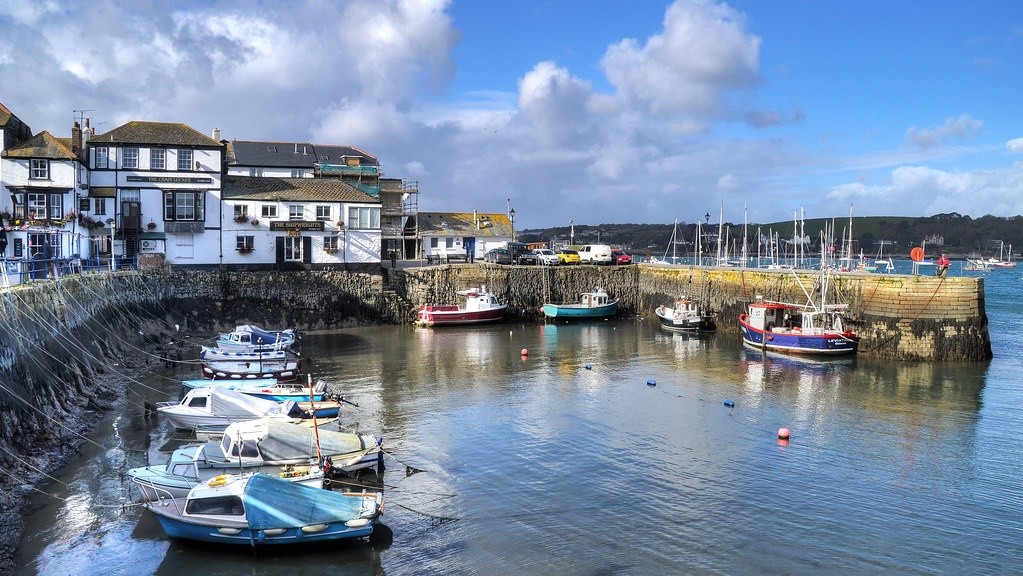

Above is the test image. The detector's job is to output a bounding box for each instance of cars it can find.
[530,248,560,266]
[483,248,512,266]
[554,249,582,265]
[605,249,632,265]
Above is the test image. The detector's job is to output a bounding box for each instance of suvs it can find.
[507,242,537,266]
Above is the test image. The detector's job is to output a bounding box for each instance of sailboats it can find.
[961,239,1018,272]
[648,196,892,272]
[915,239,936,266]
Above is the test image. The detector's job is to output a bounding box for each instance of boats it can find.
[414,286,510,327]
[125,322,387,505]
[542,287,622,319]
[738,230,860,357]
[132,429,385,547]
[654,297,717,333]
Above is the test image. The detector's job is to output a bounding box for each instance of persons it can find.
[390,251,396,267]
[939,253,949,278]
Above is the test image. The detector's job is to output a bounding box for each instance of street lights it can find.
[704,211,710,267]
[509,208,516,267]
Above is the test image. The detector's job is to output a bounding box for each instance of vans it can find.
[576,244,613,265]
